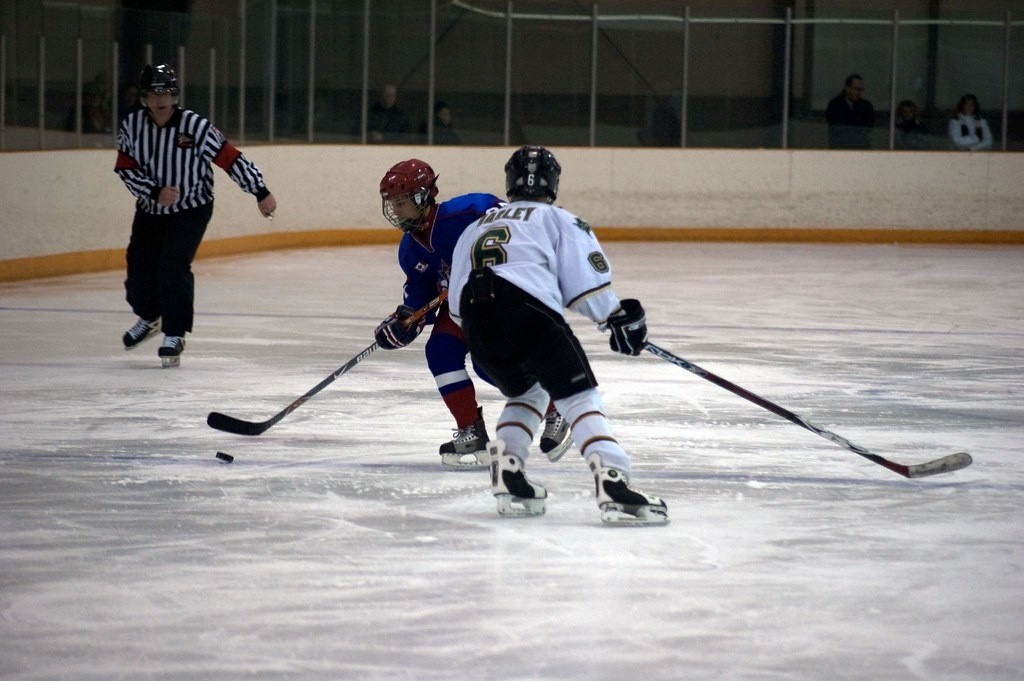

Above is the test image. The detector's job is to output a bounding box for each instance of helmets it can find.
[380,159,439,211]
[505,145,562,203]
[137,64,179,96]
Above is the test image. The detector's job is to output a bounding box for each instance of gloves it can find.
[374,304,426,351]
[607,297,648,357]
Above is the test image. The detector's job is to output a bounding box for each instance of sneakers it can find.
[485,439,548,498]
[122,317,161,347]
[538,410,571,454]
[585,452,668,513]
[158,336,186,356]
[439,406,491,455]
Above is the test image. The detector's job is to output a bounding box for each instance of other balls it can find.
[216,452,234,462]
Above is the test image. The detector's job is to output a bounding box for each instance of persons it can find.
[447,146,669,527]
[355,85,463,144]
[949,93,993,151]
[114,64,277,368]
[372,159,573,467]
[890,101,930,149]
[827,73,874,149]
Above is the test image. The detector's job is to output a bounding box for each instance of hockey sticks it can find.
[644,343,972,478]
[206,288,448,436]
